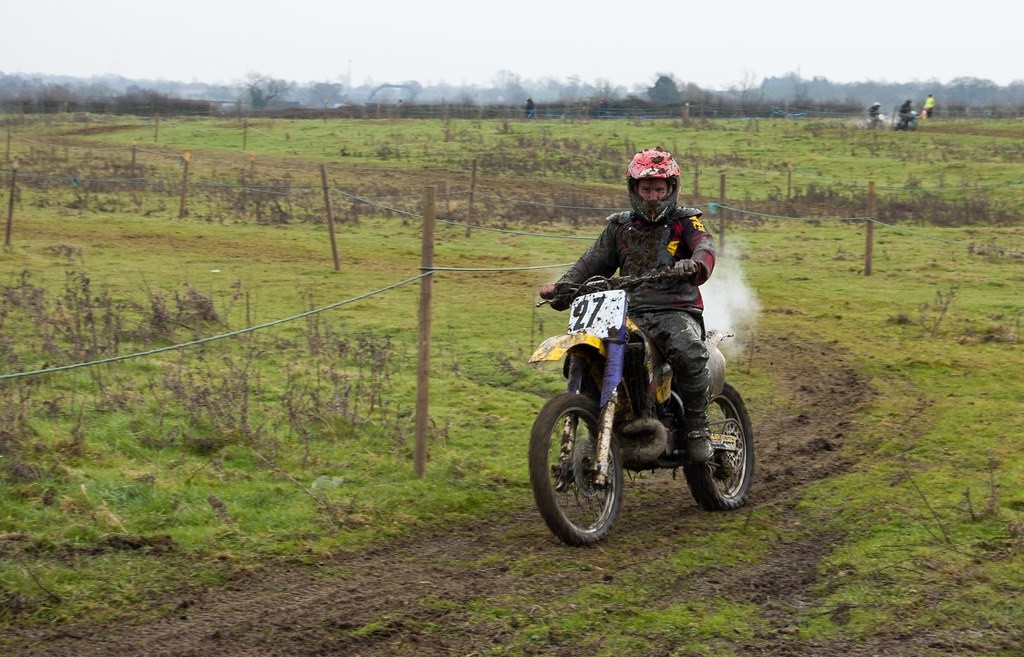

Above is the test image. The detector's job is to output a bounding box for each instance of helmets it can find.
[625,147,680,223]
[907,101,911,103]
[874,102,881,106]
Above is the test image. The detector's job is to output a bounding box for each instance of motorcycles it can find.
[893,108,917,131]
[769,104,806,118]
[866,112,889,130]
[528,265,755,545]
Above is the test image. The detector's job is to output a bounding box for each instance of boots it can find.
[685,399,715,463]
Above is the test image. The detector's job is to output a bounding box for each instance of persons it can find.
[868,102,880,122]
[599,98,609,117]
[898,100,912,126]
[539,145,716,463]
[525,98,535,118]
[923,95,935,118]
